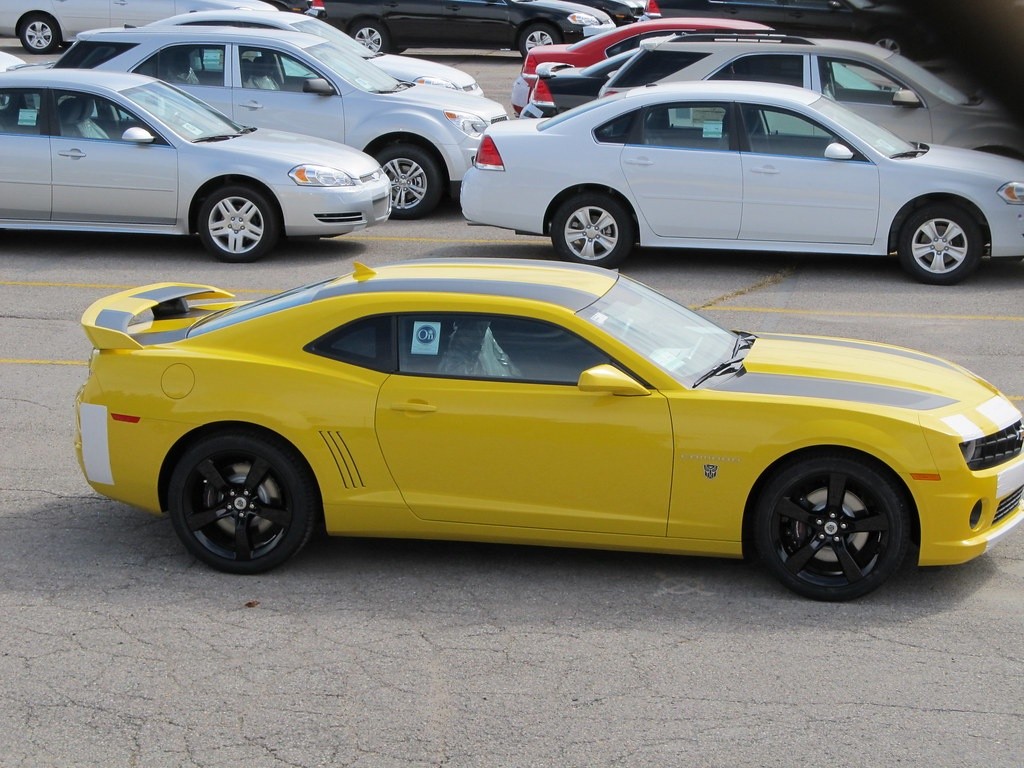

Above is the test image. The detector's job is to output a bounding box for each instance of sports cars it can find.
[74,260,1024,603]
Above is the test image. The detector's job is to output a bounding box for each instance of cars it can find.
[266,0,645,55]
[0,0,278,55]
[512,0,979,124]
[146,10,484,155]
[460,81,1024,285]
[0,69,392,263]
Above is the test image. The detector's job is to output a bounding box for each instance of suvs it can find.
[50,24,507,219]
[596,32,1024,161]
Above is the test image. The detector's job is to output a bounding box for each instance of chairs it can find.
[646,110,672,131]
[241,57,260,89]
[58,96,111,140]
